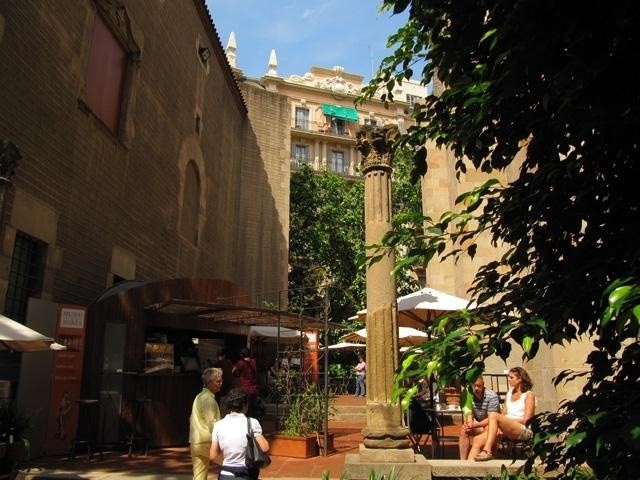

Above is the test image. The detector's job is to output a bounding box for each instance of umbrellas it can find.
[319,327,439,352]
[0,315,68,355]
[348,286,512,459]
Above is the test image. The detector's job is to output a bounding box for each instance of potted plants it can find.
[273,365,337,454]
[263,382,321,457]
[0,399,36,468]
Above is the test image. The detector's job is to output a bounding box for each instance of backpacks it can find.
[404,396,431,434]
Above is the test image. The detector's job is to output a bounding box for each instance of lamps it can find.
[199,47,211,62]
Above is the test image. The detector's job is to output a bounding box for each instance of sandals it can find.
[474,449,494,461]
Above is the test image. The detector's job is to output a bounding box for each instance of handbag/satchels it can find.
[244,434,272,468]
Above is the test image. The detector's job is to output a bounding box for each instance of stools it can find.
[124,397,152,458]
[69,399,105,463]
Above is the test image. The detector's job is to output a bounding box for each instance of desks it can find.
[425,404,463,458]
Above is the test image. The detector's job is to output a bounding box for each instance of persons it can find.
[468,367,537,461]
[208,349,233,397]
[459,374,500,459]
[209,389,269,480]
[416,372,440,405]
[189,367,223,480]
[353,355,365,397]
[232,348,258,400]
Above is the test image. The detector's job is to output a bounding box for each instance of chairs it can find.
[498,414,535,460]
[411,397,440,450]
